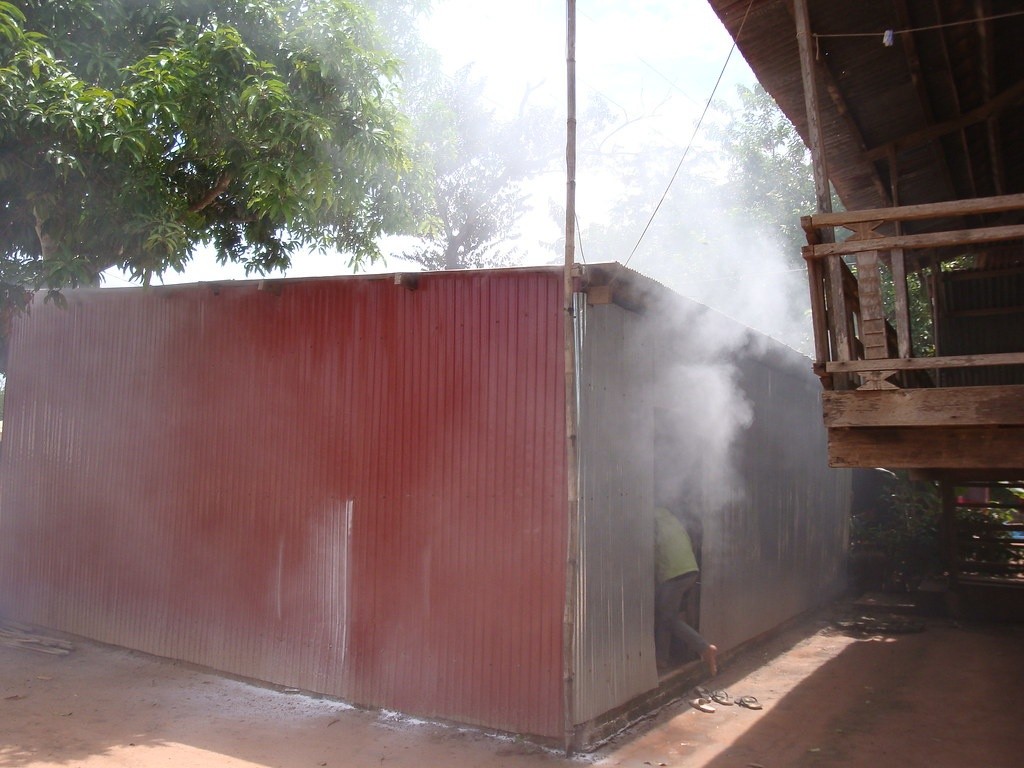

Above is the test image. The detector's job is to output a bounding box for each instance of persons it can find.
[655,507,717,678]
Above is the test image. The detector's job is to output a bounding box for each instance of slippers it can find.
[734,695,762,709]
[688,698,715,713]
[709,690,732,705]
[688,686,712,703]
